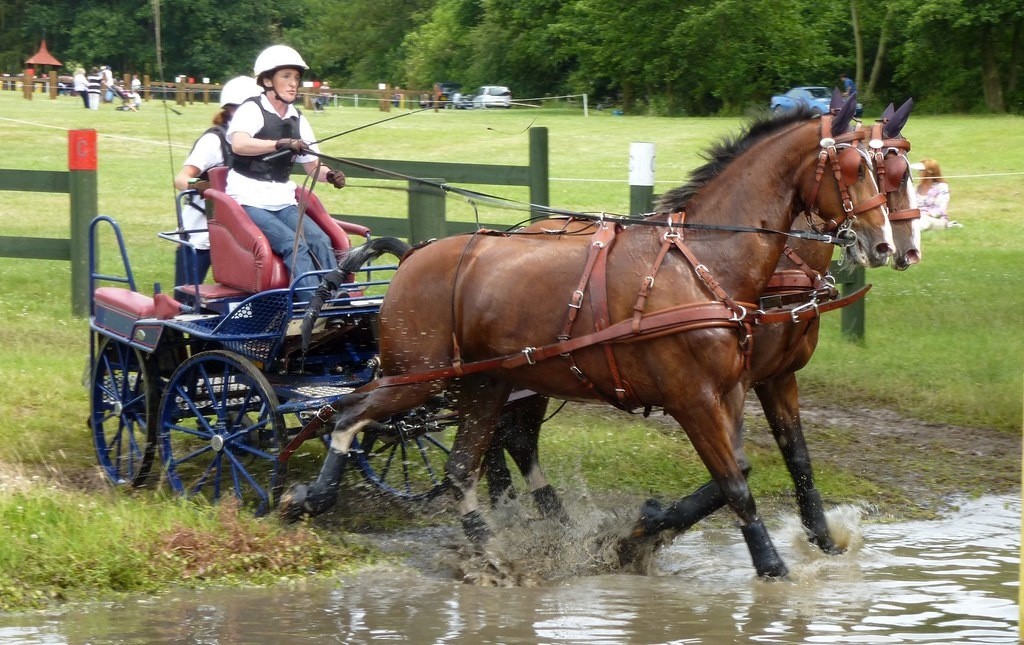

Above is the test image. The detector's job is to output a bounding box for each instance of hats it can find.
[911,163,934,174]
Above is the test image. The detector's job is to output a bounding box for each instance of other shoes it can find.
[327,292,349,307]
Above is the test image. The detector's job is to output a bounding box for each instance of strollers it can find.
[105,82,139,111]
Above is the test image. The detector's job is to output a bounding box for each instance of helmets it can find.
[220,76,265,110]
[253,44,309,89]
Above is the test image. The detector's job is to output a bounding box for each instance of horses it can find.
[271,85,924,580]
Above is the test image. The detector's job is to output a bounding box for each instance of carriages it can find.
[87,86,923,590]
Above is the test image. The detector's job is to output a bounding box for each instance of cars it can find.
[471,85,513,109]
[769,85,863,119]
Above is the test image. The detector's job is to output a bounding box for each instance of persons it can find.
[840,73,855,99]
[226,45,351,309]
[174,76,266,311]
[910,160,950,230]
[72,65,440,112]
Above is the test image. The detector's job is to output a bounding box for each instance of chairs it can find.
[204,166,355,295]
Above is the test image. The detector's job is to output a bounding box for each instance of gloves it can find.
[187,178,208,193]
[275,138,310,157]
[326,169,346,189]
